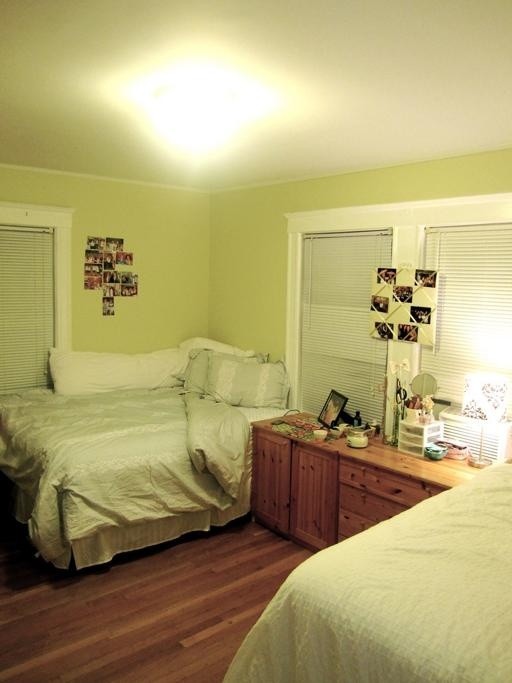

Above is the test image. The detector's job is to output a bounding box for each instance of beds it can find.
[0,337,294,569]
[223,457,512,683]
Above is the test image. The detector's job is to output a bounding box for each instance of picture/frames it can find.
[317,388,347,428]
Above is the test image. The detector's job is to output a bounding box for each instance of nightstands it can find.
[337,436,480,548]
[249,412,339,554]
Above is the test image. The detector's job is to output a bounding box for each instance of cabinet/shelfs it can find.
[397,418,444,459]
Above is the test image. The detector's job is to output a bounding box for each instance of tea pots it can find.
[345,427,373,448]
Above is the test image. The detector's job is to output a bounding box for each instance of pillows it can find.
[203,356,292,410]
[181,347,264,400]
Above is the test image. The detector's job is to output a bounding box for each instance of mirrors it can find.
[409,372,438,400]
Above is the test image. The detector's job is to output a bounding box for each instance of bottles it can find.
[353,410,362,427]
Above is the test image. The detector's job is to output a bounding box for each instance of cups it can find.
[338,424,349,433]
[313,429,328,442]
[330,426,343,438]
